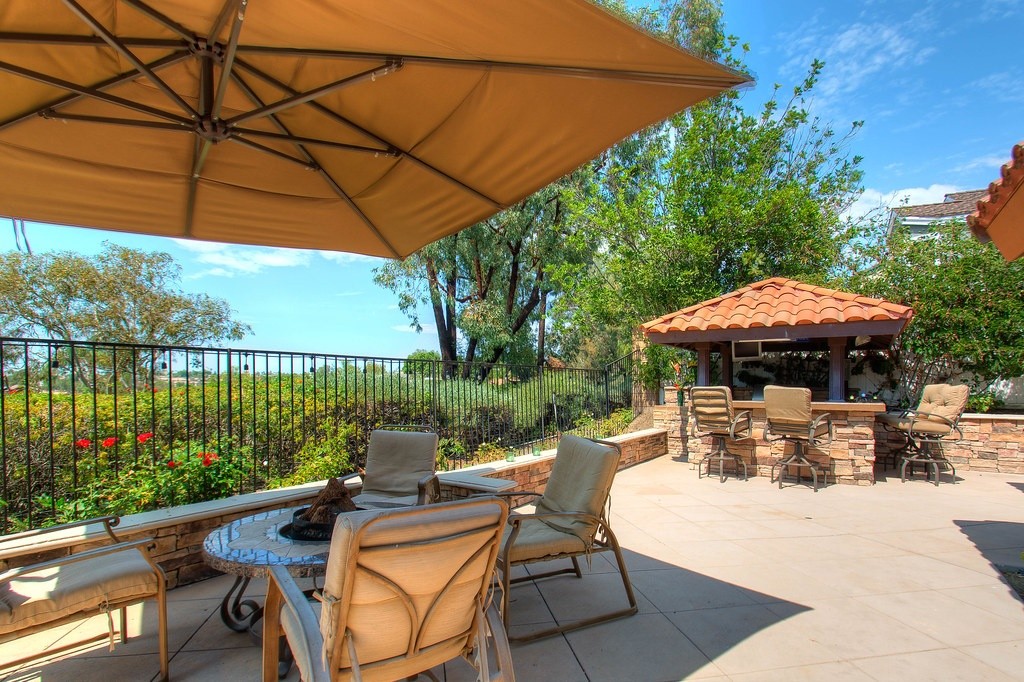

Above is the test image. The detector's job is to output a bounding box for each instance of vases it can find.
[677,390,684,406]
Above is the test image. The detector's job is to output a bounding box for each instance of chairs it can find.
[0,516,170,682]
[339,424,441,510]
[259,496,520,682]
[762,384,833,492]
[689,386,752,483]
[488,435,639,644]
[878,383,971,486]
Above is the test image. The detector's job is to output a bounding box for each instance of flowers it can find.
[670,359,693,392]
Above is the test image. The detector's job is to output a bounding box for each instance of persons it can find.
[673,363,686,406]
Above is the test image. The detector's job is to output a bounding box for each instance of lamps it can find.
[855,335,871,346]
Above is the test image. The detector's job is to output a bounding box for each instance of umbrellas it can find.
[0,2,757,263]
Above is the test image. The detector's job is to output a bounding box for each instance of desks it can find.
[204,505,377,681]
